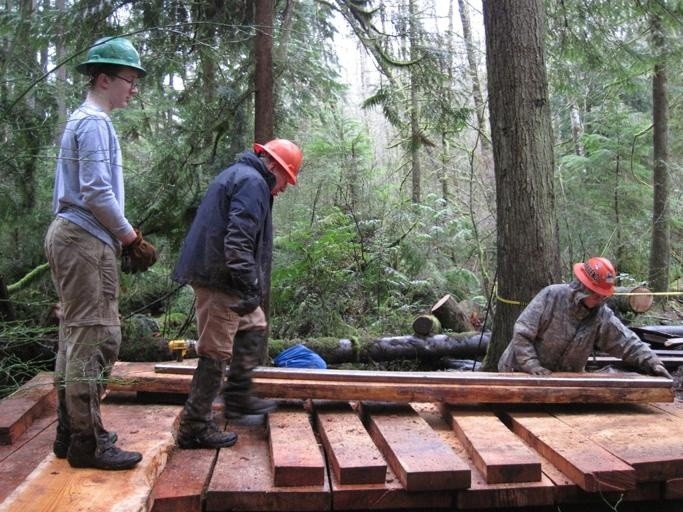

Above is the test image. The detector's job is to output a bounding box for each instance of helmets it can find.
[252,138,303,186]
[572,257,616,298]
[76,37,147,80]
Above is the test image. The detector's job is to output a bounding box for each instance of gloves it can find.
[228,289,260,318]
[529,367,552,376]
[651,364,672,380]
[120,235,156,274]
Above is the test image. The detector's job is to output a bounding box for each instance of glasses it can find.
[117,75,138,89]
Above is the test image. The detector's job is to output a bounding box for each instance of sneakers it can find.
[223,396,278,420]
[51,432,117,459]
[66,446,142,469]
[173,429,238,451]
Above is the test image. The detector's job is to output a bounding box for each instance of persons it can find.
[42,36,158,470]
[168,138,304,450]
[496,256,675,380]
